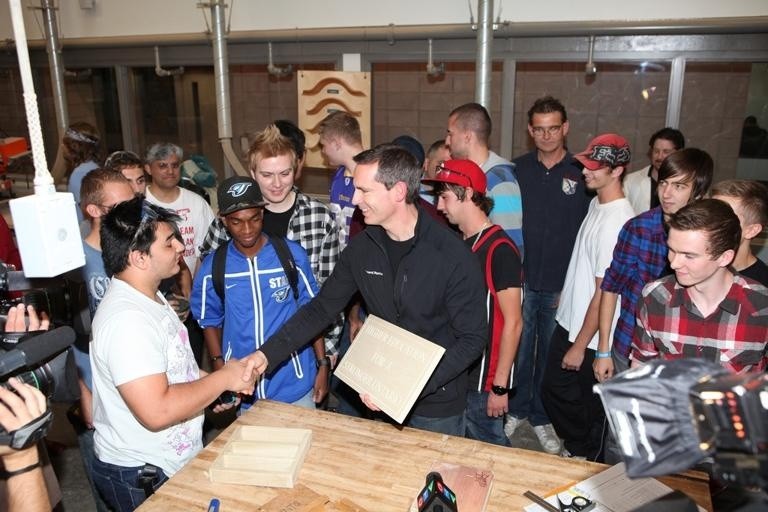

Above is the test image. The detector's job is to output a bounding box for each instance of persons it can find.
[5,300,50,335]
[0,377,54,512]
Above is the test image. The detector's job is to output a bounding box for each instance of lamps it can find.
[8,1,87,279]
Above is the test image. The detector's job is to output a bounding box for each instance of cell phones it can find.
[136,473,159,497]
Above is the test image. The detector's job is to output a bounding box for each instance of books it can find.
[524,461,710,512]
[441,461,494,512]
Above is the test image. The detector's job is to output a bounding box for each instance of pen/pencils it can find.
[524,493,557,512]
[527,490,561,512]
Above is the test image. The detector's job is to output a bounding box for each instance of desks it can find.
[134,396,765,512]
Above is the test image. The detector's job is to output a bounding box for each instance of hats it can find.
[419,159,488,195]
[575,131,631,170]
[218,176,268,213]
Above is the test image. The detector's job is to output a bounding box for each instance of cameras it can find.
[1,330,84,449]
[0,261,50,330]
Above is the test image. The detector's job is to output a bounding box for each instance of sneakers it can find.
[535,424,561,453]
[503,412,527,438]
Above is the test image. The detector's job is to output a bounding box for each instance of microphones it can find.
[0,325,77,376]
[415,471,458,512]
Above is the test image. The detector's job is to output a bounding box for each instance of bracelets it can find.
[4,459,47,480]
